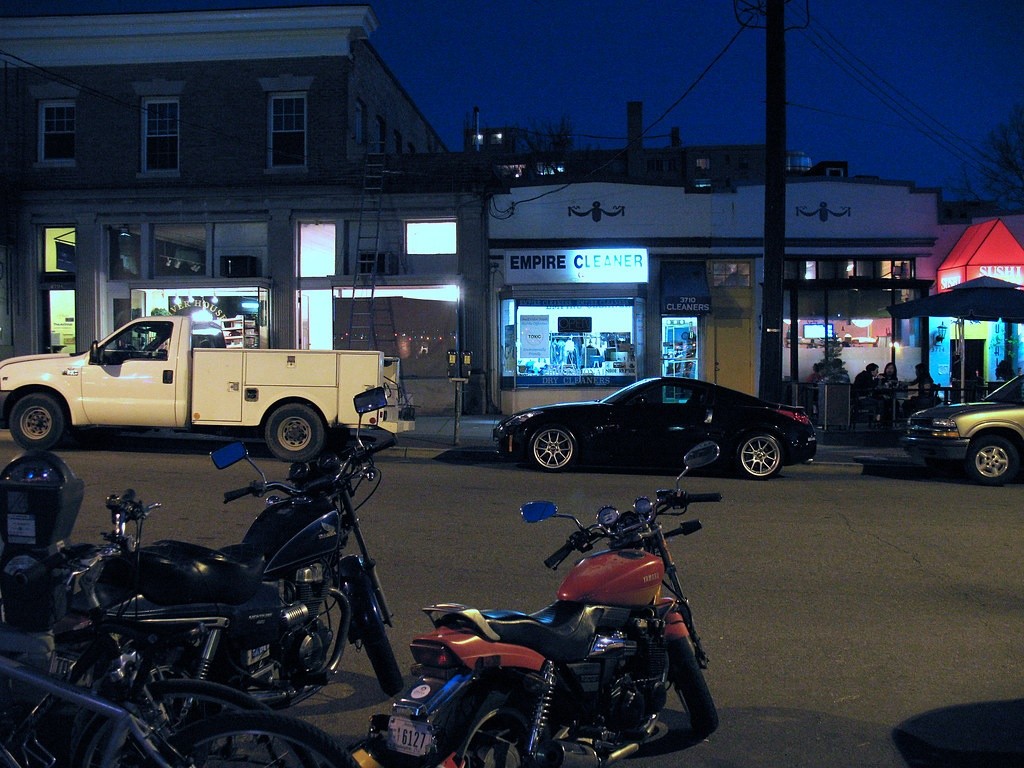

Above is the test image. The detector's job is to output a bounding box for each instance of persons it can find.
[851,364,885,419]
[806,358,850,414]
[839,341,850,347]
[873,362,900,415]
[901,363,934,421]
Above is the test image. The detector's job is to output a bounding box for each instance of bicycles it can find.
[1,486,367,768]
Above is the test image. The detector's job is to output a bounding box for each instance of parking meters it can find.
[446,348,473,446]
[0,447,88,564]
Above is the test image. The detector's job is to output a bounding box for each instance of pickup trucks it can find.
[0,316,418,464]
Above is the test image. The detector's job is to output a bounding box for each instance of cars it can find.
[492,375,819,481]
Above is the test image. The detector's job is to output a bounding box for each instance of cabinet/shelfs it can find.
[219,315,257,347]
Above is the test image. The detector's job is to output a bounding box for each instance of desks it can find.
[874,388,918,429]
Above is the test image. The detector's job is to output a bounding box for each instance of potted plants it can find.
[810,332,854,433]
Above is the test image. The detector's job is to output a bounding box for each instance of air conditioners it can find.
[359,252,398,275]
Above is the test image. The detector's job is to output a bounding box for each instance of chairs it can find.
[908,383,941,409]
[849,386,874,431]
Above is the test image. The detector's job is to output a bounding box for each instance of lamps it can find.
[166,257,200,272]
[934,322,947,346]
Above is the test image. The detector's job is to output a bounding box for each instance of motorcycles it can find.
[0,386,404,768]
[351,437,729,767]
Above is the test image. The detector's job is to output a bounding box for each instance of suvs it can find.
[898,373,1024,486]
